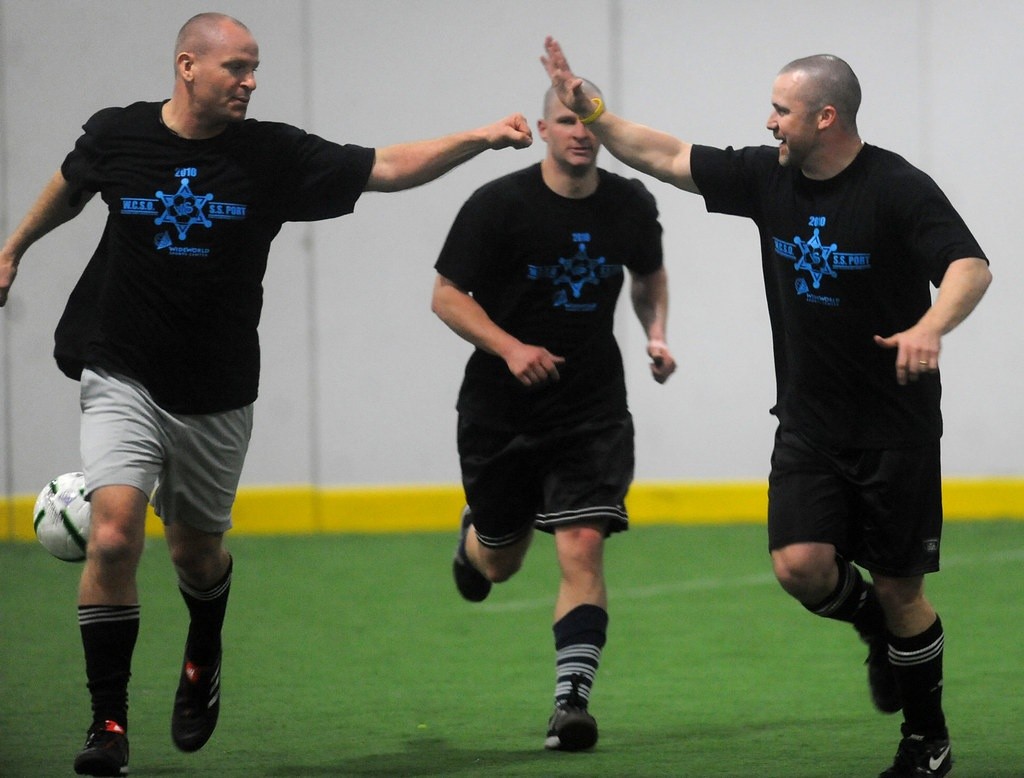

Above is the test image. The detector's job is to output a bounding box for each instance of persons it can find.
[429,73,677,755]
[540,33,997,778]
[1,9,535,775]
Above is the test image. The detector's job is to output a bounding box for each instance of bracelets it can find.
[578,96,606,126]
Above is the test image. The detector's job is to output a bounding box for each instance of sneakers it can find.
[172,650,222,754]
[453,504,493,603]
[878,723,952,778]
[73,718,129,778]
[543,704,599,753]
[865,638,904,714]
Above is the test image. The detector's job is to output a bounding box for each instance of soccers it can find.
[32,471,91,561]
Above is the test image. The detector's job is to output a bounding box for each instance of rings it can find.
[919,360,929,366]
